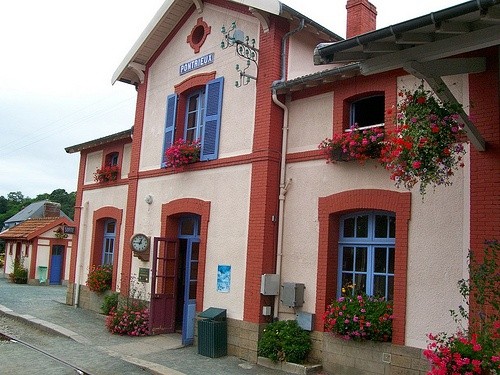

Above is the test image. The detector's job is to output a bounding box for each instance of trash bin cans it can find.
[197,307,227,358]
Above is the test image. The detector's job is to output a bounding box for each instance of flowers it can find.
[315,122,385,164]
[93,161,119,184]
[423,235,500,375]
[55,227,68,238]
[105,272,153,338]
[324,282,393,343]
[163,136,201,172]
[85,264,112,294]
[372,79,471,200]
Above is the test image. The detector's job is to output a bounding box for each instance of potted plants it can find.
[100,291,120,316]
[7,250,28,285]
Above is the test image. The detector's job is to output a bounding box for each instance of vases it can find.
[96,171,118,182]
[182,149,200,163]
[328,146,382,161]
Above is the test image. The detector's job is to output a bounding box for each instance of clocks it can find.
[129,233,151,263]
